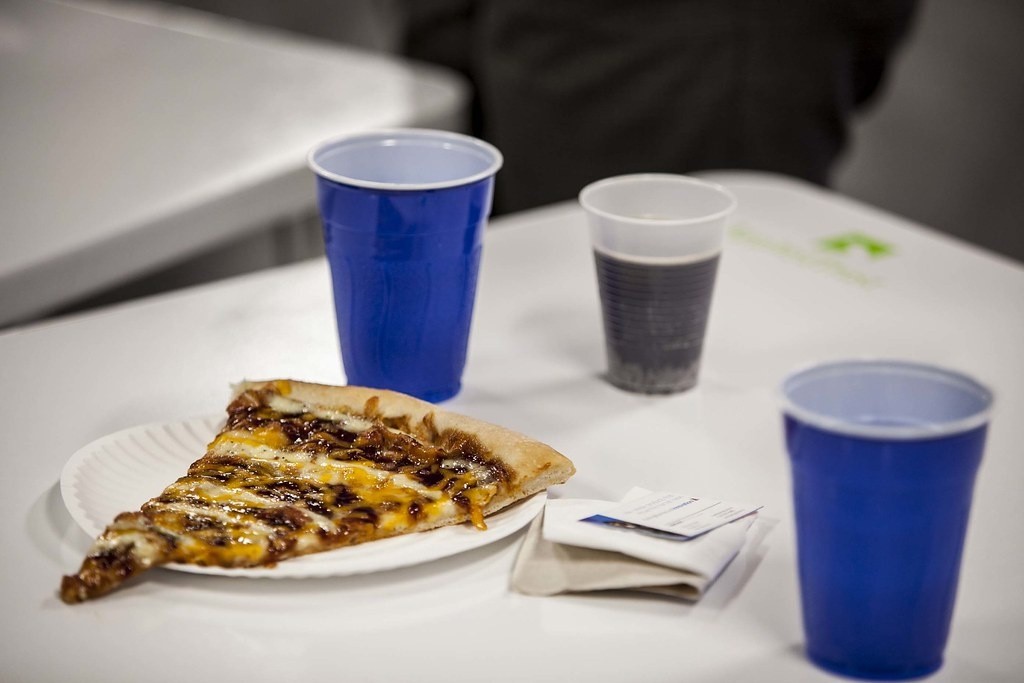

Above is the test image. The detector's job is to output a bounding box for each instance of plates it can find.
[60,415,545,580]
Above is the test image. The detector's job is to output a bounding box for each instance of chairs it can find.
[815,0,1024,265]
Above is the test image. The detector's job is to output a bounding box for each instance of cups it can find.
[771,351,998,679]
[578,172,737,391]
[309,127,506,405]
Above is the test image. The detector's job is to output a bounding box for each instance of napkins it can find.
[507,498,761,601]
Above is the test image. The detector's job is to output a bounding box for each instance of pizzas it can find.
[60,379,575,605]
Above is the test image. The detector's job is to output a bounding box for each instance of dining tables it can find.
[0,171,1024,682]
[0,0,475,329]
